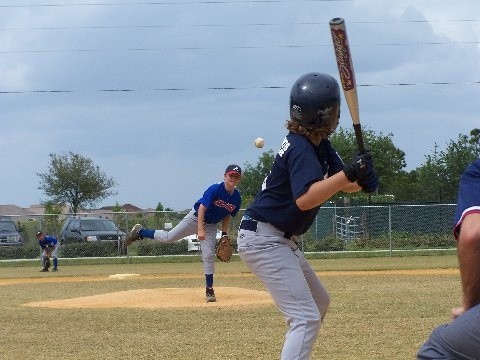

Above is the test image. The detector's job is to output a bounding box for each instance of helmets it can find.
[289,71,340,128]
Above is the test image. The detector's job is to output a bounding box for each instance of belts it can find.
[194,212,218,224]
[241,220,293,239]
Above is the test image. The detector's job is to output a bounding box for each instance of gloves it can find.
[343,149,373,182]
[358,171,379,194]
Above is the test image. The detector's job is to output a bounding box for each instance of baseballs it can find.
[255,138,264,148]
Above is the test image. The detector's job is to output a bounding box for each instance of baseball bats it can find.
[329,17,366,155]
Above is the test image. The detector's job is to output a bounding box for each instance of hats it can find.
[225,164,241,177]
[36,230,42,236]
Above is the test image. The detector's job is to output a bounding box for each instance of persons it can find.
[36,231,60,272]
[236,71,380,360]
[125,164,242,302]
[418,155,479,360]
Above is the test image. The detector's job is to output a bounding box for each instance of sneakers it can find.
[205,287,216,302]
[40,268,48,272]
[51,267,59,272]
[125,223,144,246]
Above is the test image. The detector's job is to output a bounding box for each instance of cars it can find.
[59,216,127,253]
[0,220,22,247]
[175,229,222,252]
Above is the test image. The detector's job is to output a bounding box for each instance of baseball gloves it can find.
[216,236,233,263]
